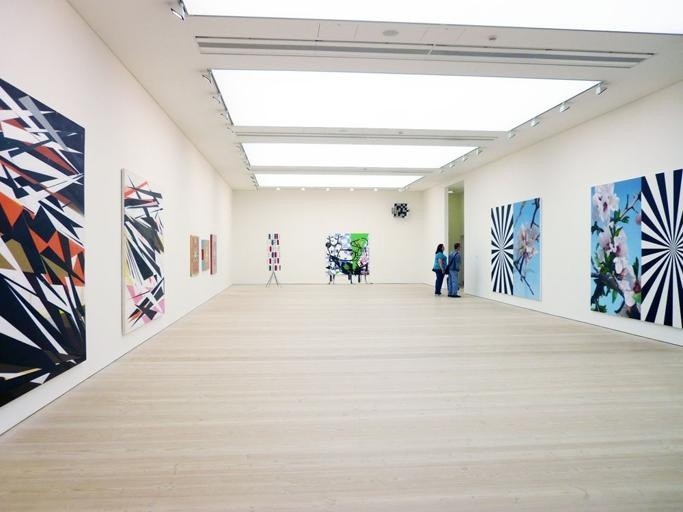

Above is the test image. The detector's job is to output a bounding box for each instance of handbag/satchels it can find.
[444,265,449,274]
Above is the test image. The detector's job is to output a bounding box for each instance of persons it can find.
[445,243,461,297]
[432,244,447,295]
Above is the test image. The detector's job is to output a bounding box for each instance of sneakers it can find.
[435,292,441,294]
[448,295,461,297]
